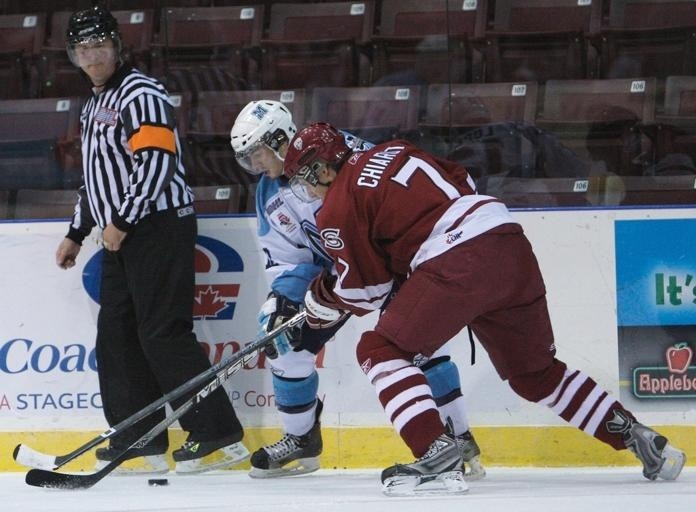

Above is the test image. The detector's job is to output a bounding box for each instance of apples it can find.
[666,342,693,373]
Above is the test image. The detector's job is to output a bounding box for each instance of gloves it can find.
[302,270,352,335]
[253,294,302,358]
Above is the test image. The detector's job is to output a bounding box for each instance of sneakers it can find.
[172,422,245,462]
[94,437,170,462]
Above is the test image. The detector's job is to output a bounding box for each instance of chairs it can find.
[0,0,696,222]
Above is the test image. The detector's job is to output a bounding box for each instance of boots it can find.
[380,415,482,484]
[605,408,669,482]
[248,398,327,470]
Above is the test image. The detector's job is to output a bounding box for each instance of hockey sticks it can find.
[26,346,265,489]
[13,311,308,470]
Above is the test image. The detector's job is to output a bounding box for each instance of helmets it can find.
[63,5,121,45]
[282,120,353,180]
[229,99,299,153]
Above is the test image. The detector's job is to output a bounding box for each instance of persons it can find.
[584,102,694,203]
[52,5,246,465]
[228,99,484,471]
[442,91,583,206]
[281,120,672,487]
